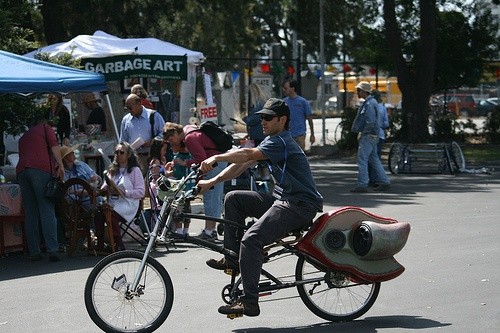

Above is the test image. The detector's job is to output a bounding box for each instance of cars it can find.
[472,98,499,118]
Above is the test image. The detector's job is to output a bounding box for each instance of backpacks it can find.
[182,121,232,152]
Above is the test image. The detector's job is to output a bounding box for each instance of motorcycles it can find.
[220,117,272,198]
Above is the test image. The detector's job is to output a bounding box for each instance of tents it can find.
[0,47,106,95]
[25,30,208,125]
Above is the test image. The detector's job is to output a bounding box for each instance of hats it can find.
[60,146,74,160]
[81,93,101,104]
[354,81,373,94]
[255,97,290,115]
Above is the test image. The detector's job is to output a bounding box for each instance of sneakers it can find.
[207,257,240,273]
[218,297,260,316]
[194,231,218,242]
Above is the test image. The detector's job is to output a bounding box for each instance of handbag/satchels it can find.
[47,175,63,198]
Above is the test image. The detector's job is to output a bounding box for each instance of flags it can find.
[224,64,376,84]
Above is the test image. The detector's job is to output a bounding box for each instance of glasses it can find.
[123,103,137,110]
[114,150,129,155]
[259,115,279,121]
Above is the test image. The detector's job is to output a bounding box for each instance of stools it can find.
[108,214,149,251]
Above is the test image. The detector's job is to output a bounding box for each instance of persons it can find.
[195,98,321,317]
[16,84,229,249]
[243,83,275,188]
[349,80,392,193]
[280,78,317,150]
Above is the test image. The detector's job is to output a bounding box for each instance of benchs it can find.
[374,141,451,172]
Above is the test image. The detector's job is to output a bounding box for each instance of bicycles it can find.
[385,136,497,180]
[83,160,409,333]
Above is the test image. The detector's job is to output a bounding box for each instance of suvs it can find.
[429,94,478,119]
